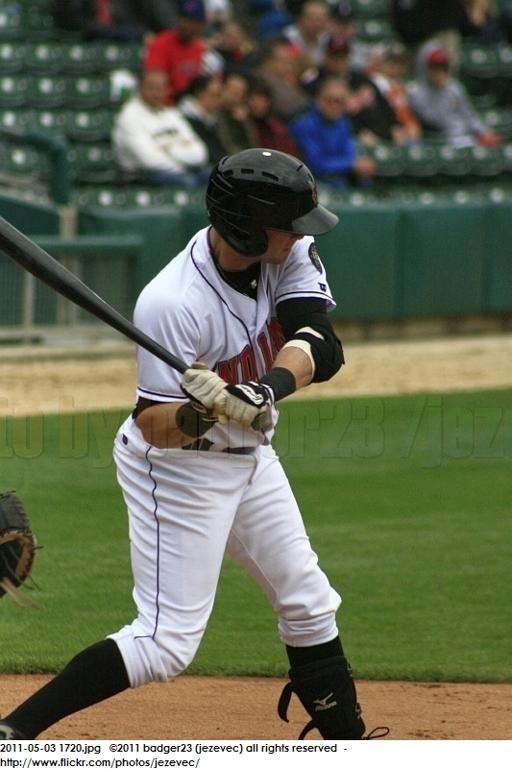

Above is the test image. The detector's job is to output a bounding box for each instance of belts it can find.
[181,438,257,456]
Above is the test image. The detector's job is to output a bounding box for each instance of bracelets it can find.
[175,403,217,439]
[257,367,297,402]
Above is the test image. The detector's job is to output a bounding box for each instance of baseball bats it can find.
[0,218,270,430]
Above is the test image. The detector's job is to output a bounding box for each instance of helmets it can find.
[205,148,339,259]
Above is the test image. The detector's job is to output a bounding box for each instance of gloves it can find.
[213,380,280,430]
[178,362,226,427]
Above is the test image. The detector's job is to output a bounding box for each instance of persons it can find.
[52,1,511,192]
[0,145,390,739]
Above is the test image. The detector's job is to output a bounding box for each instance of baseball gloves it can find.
[1,492,47,611]
